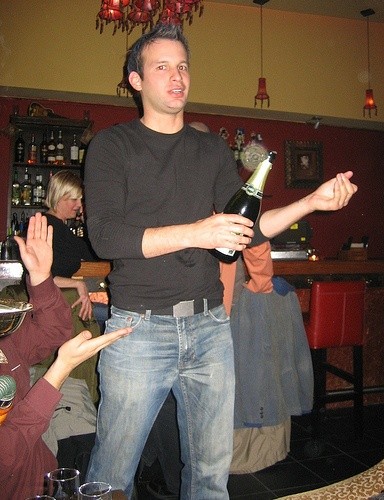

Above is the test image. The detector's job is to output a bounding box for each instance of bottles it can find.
[49,170,52,179]
[40,173,45,206]
[20,172,32,206]
[79,143,84,164]
[32,174,42,206]
[28,134,38,163]
[70,134,79,164]
[2,237,12,261]
[15,132,25,163]
[47,129,55,165]
[69,212,84,238]
[205,151,277,265]
[40,130,47,165]
[56,131,65,165]
[12,172,20,206]
[11,212,30,237]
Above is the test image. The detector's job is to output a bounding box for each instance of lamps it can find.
[254,0,270,106]
[96,0,203,35]
[362,9,377,117]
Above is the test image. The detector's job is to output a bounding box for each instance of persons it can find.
[82,23,359,500]
[154,212,273,495]
[42,171,92,321]
[0,212,133,500]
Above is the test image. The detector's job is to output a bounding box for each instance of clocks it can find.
[285,140,323,189]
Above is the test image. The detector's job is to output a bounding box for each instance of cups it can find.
[78,481,113,500]
[25,494,55,500]
[47,468,81,500]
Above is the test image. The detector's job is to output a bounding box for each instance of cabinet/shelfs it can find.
[7,115,93,243]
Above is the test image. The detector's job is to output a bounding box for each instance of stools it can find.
[304,281,364,421]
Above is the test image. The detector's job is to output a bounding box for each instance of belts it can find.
[121,296,224,319]
